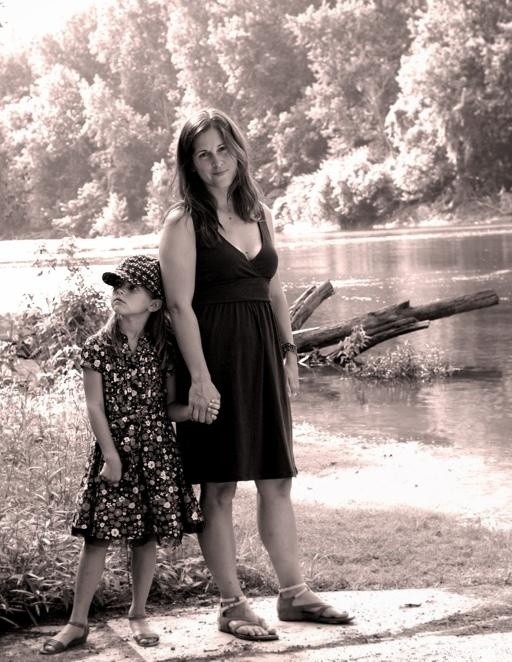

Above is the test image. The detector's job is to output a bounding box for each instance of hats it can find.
[102,256,164,299]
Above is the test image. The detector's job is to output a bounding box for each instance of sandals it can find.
[277,582,356,623]
[218,596,278,640]
[41,622,89,655]
[128,614,159,646]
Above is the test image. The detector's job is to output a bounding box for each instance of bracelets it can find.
[280,341,299,359]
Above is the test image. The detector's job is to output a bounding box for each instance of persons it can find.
[157,105,356,642]
[38,256,221,655]
